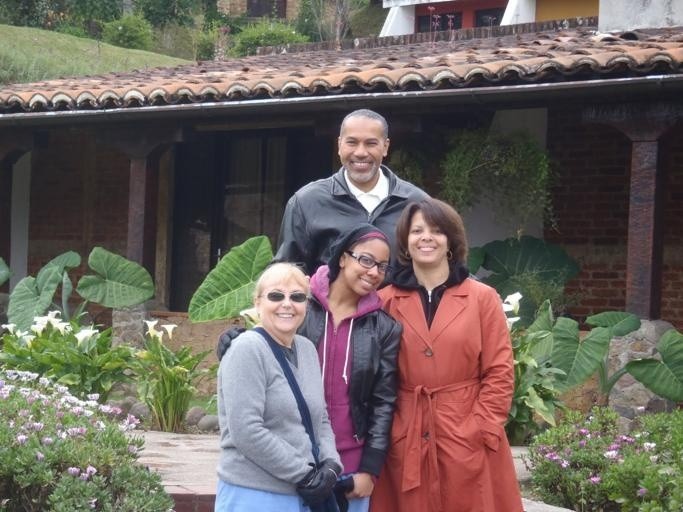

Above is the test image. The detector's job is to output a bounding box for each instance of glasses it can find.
[344,248,394,275]
[258,291,307,302]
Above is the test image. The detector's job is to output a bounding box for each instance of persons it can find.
[215,223,402,512]
[362,195,524,512]
[215,106,433,363]
[213,263,343,512]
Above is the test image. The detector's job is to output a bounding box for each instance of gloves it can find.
[295,459,355,512]
[216,326,247,362]
